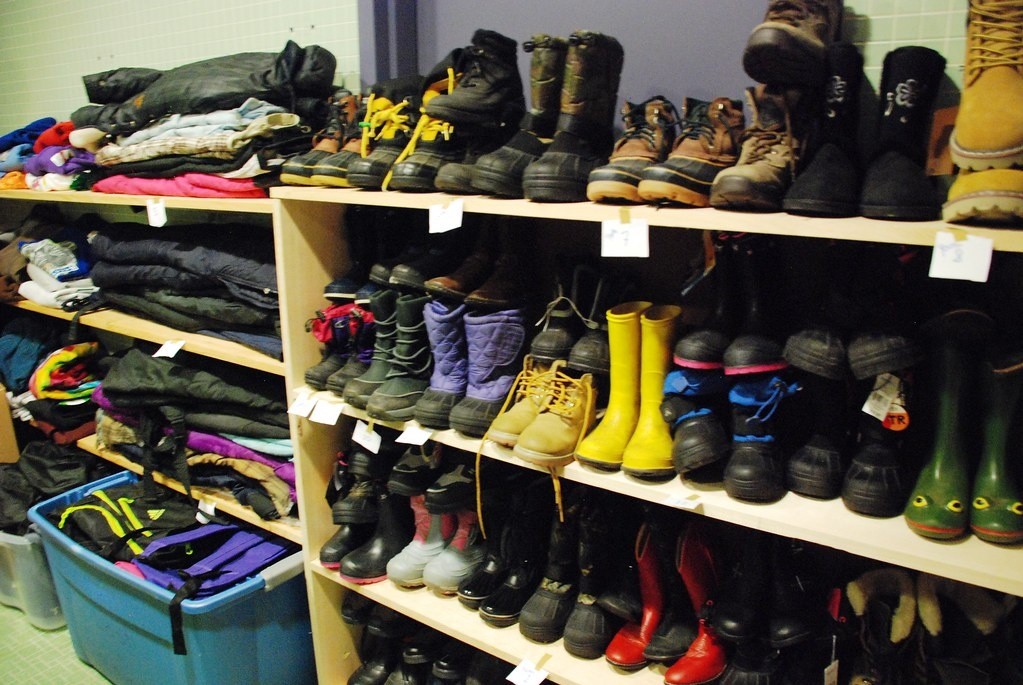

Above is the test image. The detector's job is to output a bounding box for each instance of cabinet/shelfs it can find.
[274,178,1023,685]
[0,187,304,547]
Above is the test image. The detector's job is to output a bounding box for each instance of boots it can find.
[281,0,1022,684]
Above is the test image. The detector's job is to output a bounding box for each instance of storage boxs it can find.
[0,456,121,632]
[23,472,315,684]
